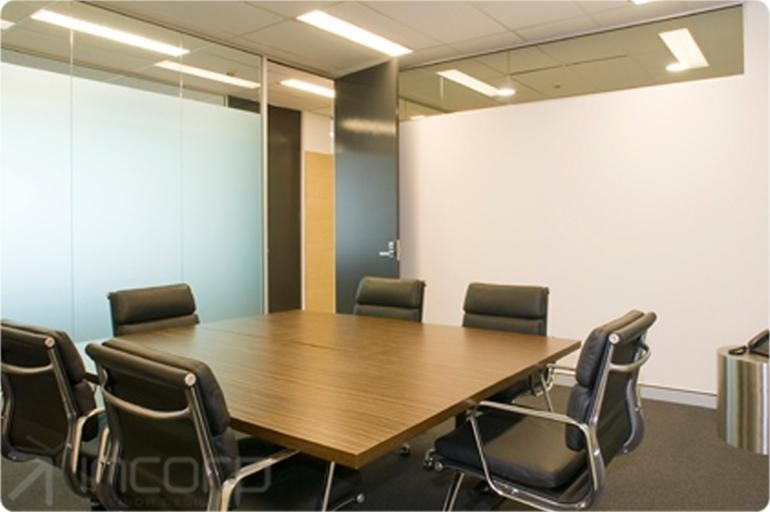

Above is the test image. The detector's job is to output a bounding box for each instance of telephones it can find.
[749,330,769,356]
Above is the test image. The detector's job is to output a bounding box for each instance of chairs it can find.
[109,282,200,336]
[85,339,366,511]
[1,319,110,497]
[423,282,554,470]
[353,275,426,458]
[433,310,656,510]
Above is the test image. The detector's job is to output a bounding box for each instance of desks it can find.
[116,308,582,511]
